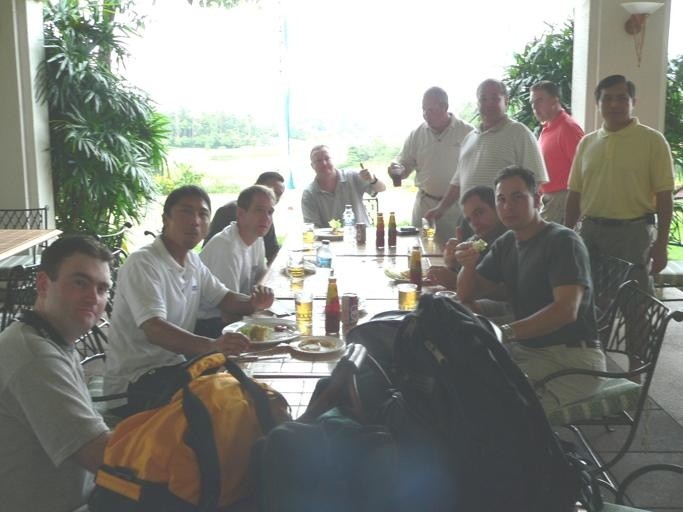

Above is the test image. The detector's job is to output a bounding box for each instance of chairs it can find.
[590,249,636,351]
[0,204,50,328]
[598,462,682,512]
[361,198,380,226]
[70,223,136,366]
[531,279,683,475]
[4,262,40,326]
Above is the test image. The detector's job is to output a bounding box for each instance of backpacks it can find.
[394,296,585,511]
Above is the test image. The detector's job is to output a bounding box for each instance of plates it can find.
[220,317,310,345]
[313,228,344,238]
[288,335,346,354]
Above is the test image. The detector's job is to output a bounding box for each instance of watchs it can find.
[372,173,377,186]
[500,325,516,343]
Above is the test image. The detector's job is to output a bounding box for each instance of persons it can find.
[389,88,479,230]
[430,186,504,289]
[1,236,112,512]
[424,80,544,228]
[104,186,273,415]
[457,167,606,416]
[302,146,387,229]
[566,76,674,385]
[529,82,589,226]
[199,186,275,337]
[203,173,284,262]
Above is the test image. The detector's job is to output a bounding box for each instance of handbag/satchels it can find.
[86,351,294,512]
[250,342,464,512]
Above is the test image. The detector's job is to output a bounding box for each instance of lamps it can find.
[619,2,669,67]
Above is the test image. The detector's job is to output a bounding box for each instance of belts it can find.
[585,213,656,227]
[520,338,604,350]
[418,188,445,201]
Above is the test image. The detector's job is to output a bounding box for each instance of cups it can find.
[396,282,418,311]
[302,223,315,245]
[294,291,313,321]
[422,216,436,238]
[286,251,305,277]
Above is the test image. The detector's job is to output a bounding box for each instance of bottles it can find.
[343,205,356,250]
[325,278,340,333]
[389,213,396,247]
[356,223,366,243]
[341,293,359,327]
[410,246,422,291]
[376,213,384,248]
[315,240,332,296]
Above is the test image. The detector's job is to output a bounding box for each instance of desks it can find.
[0,228,63,261]
